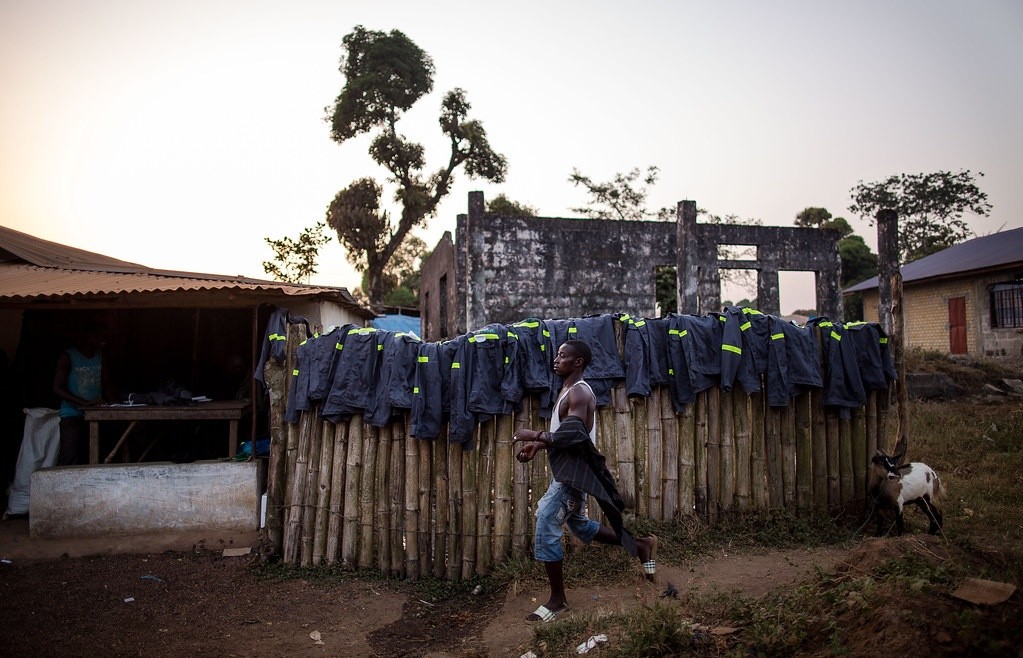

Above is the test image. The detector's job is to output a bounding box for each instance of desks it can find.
[85,401,251,465]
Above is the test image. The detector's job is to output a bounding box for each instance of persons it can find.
[53,320,123,464]
[509,340,658,624]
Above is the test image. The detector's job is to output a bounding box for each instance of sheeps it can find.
[866,453,948,538]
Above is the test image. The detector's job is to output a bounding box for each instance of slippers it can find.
[642,534,656,583]
[524,605,570,624]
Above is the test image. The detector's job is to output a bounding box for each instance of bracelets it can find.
[536,431,546,440]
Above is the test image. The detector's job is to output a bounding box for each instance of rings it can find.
[513,436,517,440]
